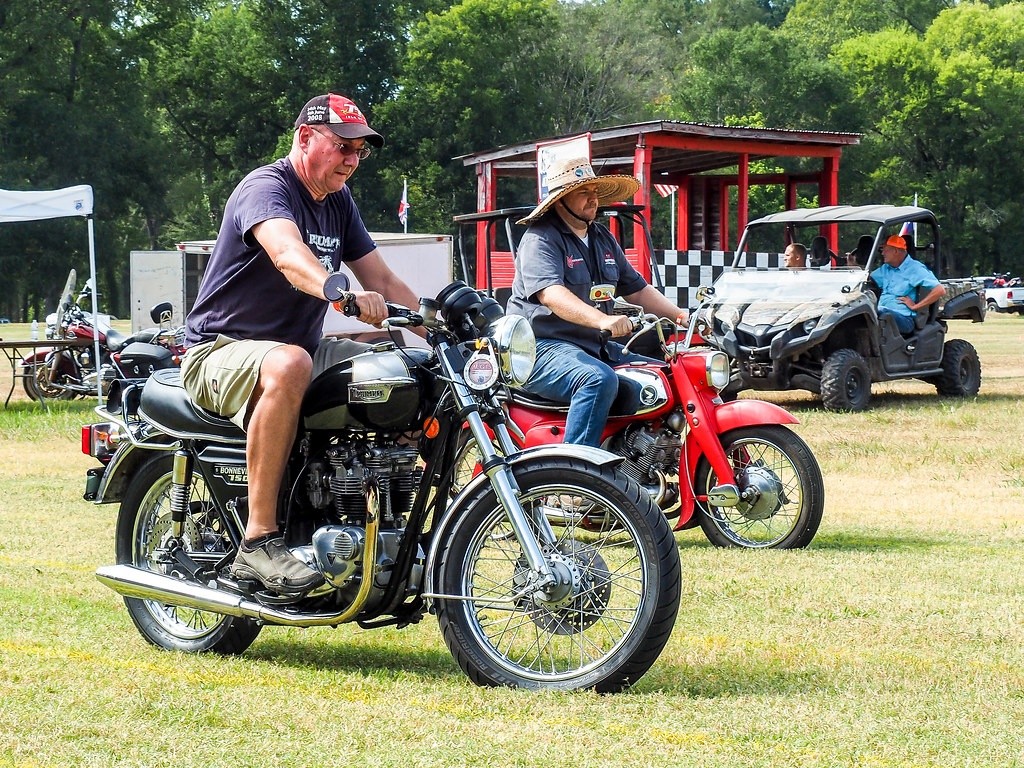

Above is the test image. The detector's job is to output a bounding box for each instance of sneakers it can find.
[547,493,591,512]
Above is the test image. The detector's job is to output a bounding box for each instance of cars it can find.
[0,318,9,325]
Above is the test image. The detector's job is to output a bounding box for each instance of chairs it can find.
[856,235,876,266]
[810,237,832,266]
[495,287,512,316]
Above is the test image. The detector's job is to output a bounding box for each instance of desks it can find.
[3,340,88,415]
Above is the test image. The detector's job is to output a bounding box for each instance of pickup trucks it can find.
[969,275,1024,315]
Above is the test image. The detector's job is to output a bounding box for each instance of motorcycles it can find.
[78,268,685,701]
[448,284,826,555]
[18,268,188,402]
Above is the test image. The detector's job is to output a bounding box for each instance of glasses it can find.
[310,127,371,160]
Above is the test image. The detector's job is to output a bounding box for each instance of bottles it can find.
[31,320,38,341]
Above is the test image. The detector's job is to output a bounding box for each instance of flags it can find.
[398,179,410,225]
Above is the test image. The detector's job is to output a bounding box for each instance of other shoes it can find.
[231,537,326,593]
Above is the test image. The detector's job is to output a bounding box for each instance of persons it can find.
[505,158,710,512]
[847,235,946,334]
[993,276,1006,287]
[180,92,430,591]
[784,243,807,267]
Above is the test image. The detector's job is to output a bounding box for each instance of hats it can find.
[294,93,384,149]
[516,156,642,225]
[879,235,907,249]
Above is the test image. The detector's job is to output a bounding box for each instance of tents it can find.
[0,185,103,406]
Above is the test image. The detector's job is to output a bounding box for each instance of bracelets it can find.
[333,302,344,312]
[676,311,687,324]
